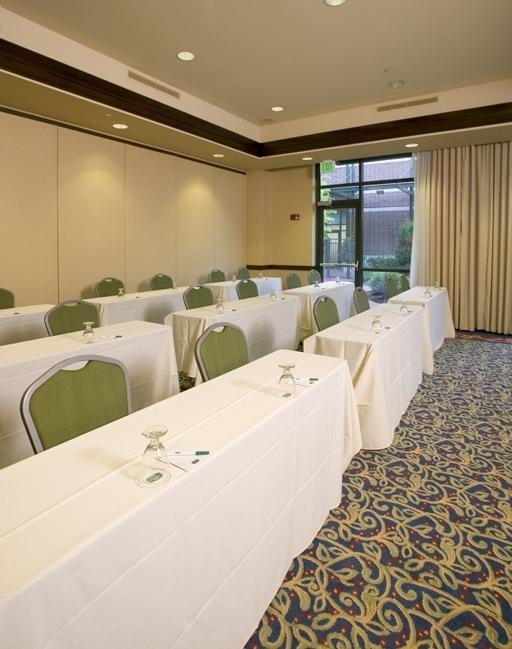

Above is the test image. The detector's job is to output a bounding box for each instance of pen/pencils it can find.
[277,298,285,301]
[398,311,413,312]
[99,335,122,338]
[170,451,210,455]
[225,309,237,311]
[297,377,319,381]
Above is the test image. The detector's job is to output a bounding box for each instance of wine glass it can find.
[118,272,264,299]
[212,272,342,314]
[136,424,171,489]
[365,272,441,337]
[277,363,298,400]
